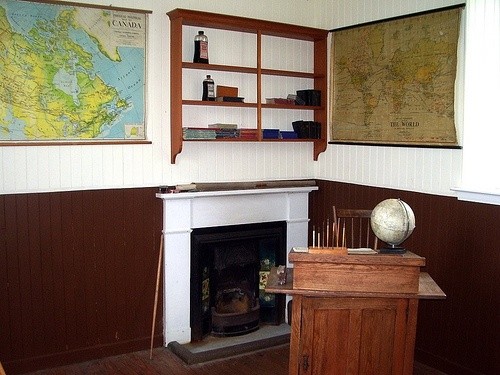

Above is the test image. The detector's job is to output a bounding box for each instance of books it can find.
[261,120,321,139]
[266,89,321,105]
[348,248,377,254]
[182,123,259,140]
[215,85,244,102]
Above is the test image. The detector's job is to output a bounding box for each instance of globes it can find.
[370,199,416,253]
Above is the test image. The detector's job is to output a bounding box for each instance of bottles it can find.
[202,75,215,101]
[193,31,209,63]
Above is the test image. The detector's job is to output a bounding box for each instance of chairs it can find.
[333,205,378,251]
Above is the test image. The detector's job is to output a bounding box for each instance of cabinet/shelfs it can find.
[166,8,327,164]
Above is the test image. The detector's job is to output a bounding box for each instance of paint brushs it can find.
[317,225,320,248]
[342,218,345,248]
[312,223,315,249]
[327,217,329,248]
[332,222,335,250]
[322,219,324,249]
[332,206,340,248]
